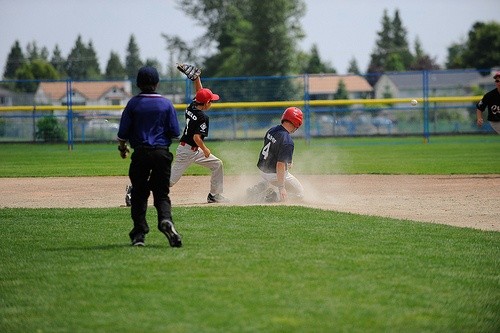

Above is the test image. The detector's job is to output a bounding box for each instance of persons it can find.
[247,107,305,205]
[125,75,230,206]
[476,70,500,136]
[116,65,182,249]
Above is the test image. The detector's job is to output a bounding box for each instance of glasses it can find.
[495,80,500,82]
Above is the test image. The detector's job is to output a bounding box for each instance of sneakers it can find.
[206,193,229,203]
[132,233,145,247]
[124,185,133,207]
[158,217,182,248]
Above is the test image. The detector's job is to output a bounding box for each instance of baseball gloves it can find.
[177,63,201,80]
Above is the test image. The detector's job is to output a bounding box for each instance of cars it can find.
[89,119,120,132]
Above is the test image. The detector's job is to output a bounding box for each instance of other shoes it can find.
[246,185,260,197]
[265,189,277,203]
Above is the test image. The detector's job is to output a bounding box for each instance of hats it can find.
[195,88,220,104]
[493,71,500,79]
[137,66,159,85]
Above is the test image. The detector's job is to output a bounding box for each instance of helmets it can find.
[281,107,304,128]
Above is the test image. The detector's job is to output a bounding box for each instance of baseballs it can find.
[410,100,417,106]
